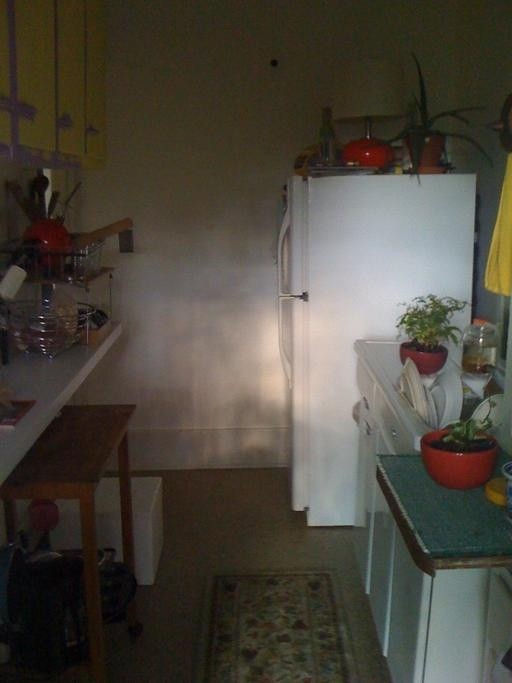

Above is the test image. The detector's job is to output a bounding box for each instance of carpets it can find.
[191,567,360,683]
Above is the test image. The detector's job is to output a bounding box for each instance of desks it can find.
[0,320,124,486]
[0,403,137,683]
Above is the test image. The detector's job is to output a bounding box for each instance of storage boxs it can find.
[0,476,163,587]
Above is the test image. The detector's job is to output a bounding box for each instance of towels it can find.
[483,152,512,297]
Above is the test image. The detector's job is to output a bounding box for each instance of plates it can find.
[398,356,504,432]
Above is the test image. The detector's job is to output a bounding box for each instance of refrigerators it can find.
[275,170,480,530]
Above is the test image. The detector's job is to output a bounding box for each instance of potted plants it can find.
[364,50,495,185]
[393,293,467,374]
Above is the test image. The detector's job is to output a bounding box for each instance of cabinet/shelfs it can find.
[351,353,512,683]
[0,0,107,172]
